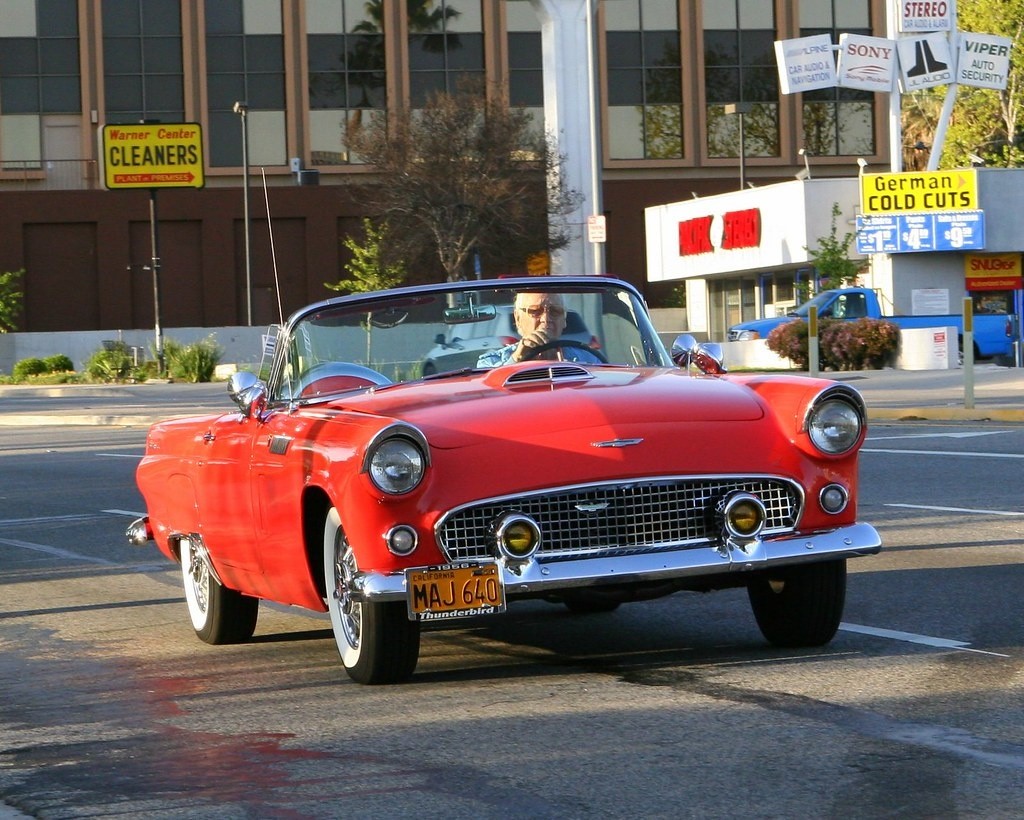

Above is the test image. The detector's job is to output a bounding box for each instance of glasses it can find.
[518,305,567,318]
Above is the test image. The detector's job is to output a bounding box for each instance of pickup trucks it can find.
[726,289,1013,361]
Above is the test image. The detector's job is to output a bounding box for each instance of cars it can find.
[417,303,605,375]
[126,275,883,681]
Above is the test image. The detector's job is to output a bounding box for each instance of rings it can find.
[522,339,524,345]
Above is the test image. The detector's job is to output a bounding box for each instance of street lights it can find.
[232,102,253,326]
[723,102,749,190]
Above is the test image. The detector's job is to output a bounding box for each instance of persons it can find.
[477,292,609,366]
[843,298,866,317]
